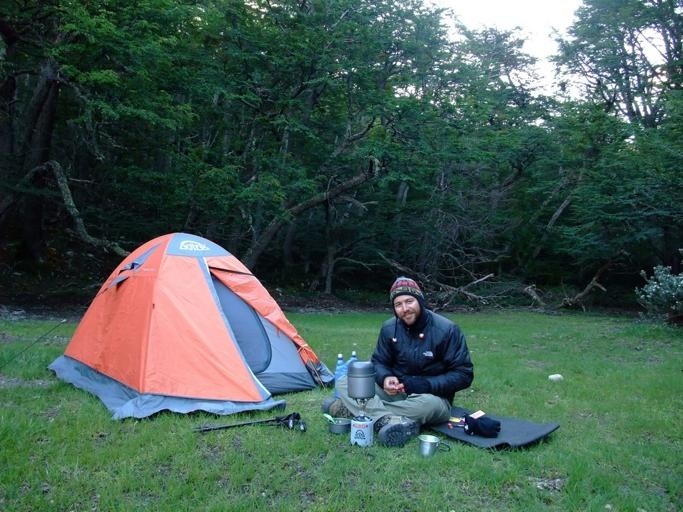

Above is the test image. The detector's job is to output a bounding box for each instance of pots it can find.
[347,360,376,398]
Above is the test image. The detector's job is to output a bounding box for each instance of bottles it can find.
[334,350,360,399]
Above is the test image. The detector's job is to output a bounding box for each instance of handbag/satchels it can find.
[464,412,501,438]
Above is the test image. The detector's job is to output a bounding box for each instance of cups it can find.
[417,435,450,458]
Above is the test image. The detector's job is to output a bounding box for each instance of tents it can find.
[44,233,335,423]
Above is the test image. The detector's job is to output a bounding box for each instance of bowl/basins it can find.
[328,418,350,433]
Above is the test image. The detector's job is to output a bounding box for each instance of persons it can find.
[321,275,474,448]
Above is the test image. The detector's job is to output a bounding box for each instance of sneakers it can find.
[378,415,420,448]
[328,400,354,418]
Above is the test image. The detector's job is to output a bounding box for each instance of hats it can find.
[390,276,424,343]
